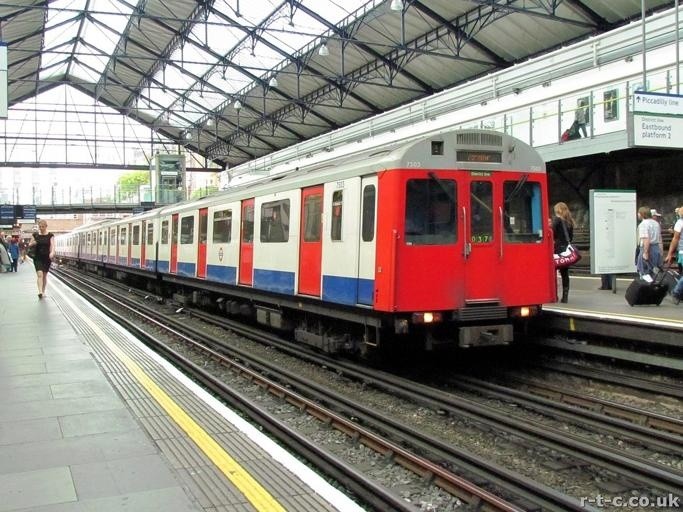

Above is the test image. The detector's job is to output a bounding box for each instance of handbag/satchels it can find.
[553,242,582,268]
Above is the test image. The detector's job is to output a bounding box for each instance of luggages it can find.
[624,261,673,308]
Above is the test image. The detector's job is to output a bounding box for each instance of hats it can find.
[650,208,661,216]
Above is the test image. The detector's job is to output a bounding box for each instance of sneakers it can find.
[38,293,43,299]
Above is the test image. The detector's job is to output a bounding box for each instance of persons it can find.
[575,100,587,138]
[637,206,683,280]
[598,274,613,290]
[0,232,28,272]
[28,219,55,299]
[553,202,574,303]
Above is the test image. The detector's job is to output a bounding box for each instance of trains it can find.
[51,129,558,376]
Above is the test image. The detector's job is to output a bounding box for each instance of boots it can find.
[561,286,568,303]
